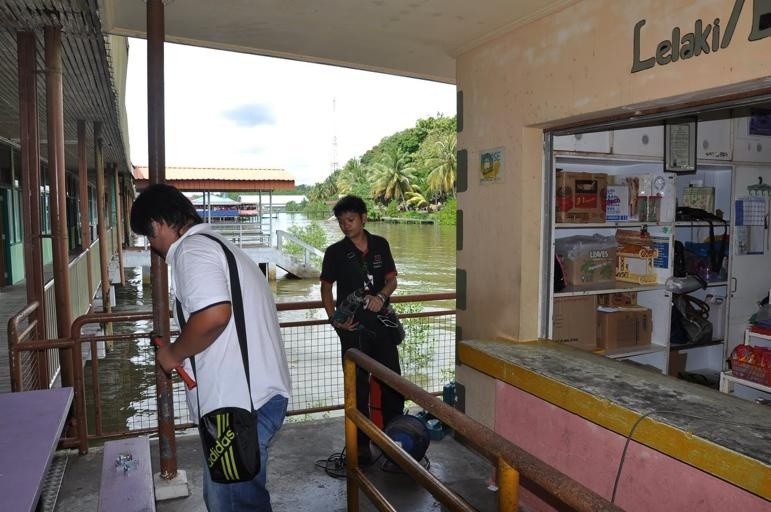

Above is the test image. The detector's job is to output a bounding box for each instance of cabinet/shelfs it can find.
[547,153,735,390]
[719,330,771,406]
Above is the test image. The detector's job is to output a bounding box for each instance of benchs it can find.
[98,437,156,512]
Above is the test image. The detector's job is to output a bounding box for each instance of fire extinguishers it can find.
[368,374,384,446]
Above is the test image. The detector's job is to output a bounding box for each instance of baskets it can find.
[729,357,771,387]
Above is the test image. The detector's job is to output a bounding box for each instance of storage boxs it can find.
[555,242,617,285]
[556,171,607,224]
[553,291,651,352]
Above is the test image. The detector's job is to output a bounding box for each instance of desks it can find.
[0,386,75,512]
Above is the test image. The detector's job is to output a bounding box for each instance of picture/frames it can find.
[664,122,698,175]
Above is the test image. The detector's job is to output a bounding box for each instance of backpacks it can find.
[674,293,714,343]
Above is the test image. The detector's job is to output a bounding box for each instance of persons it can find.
[319,194,407,472]
[129,182,295,511]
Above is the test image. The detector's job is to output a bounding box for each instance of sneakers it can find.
[357,446,371,464]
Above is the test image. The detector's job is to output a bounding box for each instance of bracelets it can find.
[377,292,387,304]
[329,316,335,324]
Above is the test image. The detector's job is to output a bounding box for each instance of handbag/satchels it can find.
[677,205,728,289]
[364,307,405,349]
[196,407,261,485]
